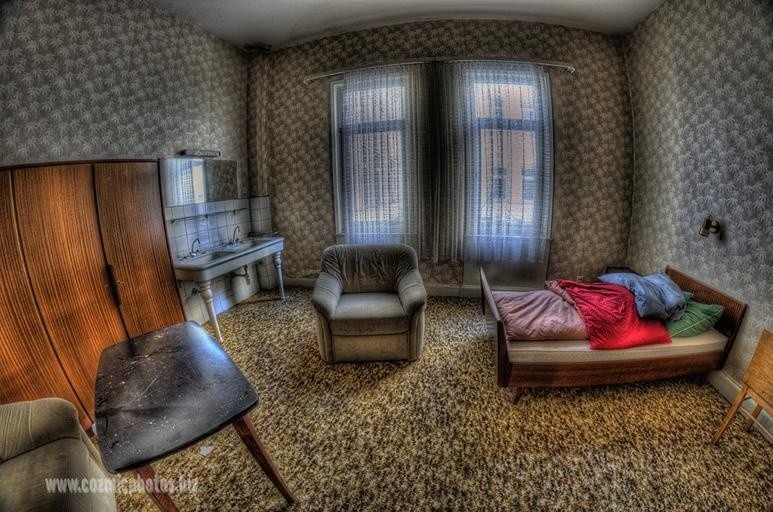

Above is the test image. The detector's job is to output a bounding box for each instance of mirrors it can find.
[163,157,237,207]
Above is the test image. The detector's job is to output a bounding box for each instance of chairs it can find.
[0,395,121,512]
[312,240,430,364]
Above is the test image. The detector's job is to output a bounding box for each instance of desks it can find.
[89,317,298,511]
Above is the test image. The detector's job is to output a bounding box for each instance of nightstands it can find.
[604,267,645,277]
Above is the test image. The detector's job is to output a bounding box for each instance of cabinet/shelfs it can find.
[11,156,187,426]
[0,166,94,446]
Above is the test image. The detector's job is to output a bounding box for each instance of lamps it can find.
[698,214,719,239]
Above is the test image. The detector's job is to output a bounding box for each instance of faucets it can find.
[231,225,241,242]
[191,237,201,252]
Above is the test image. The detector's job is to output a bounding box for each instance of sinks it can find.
[229,239,271,251]
[185,250,235,266]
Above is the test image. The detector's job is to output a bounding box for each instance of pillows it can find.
[643,272,692,310]
[665,299,724,339]
[597,272,668,321]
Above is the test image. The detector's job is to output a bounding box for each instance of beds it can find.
[473,264,749,407]
